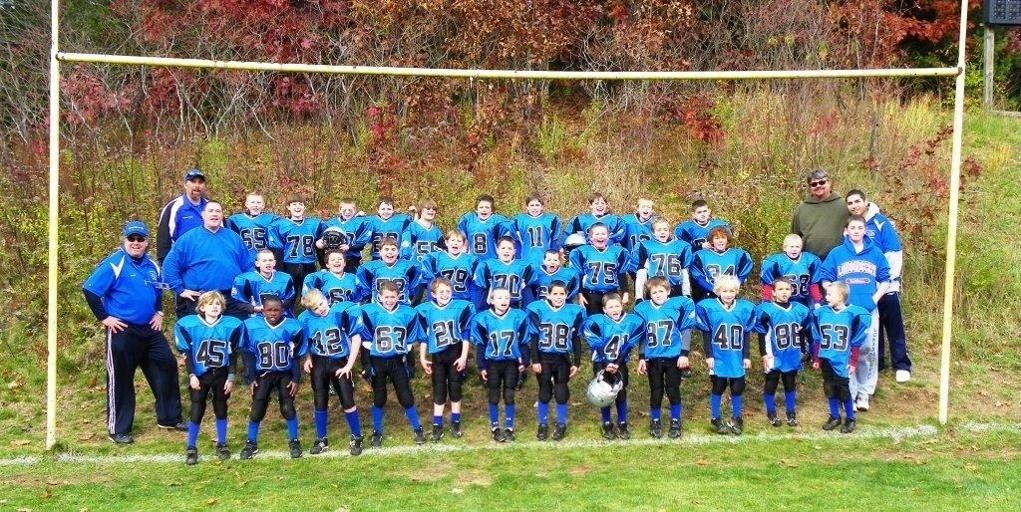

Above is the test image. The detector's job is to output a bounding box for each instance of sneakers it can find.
[371,428,383,447]
[349,433,364,455]
[414,420,462,444]
[215,442,231,461]
[240,439,259,460]
[157,421,189,431]
[490,426,506,442]
[895,369,911,383]
[601,412,855,440]
[536,423,549,441]
[107,430,134,444]
[502,427,515,443]
[856,392,870,412]
[186,448,197,465]
[842,400,858,412]
[310,437,329,454]
[288,439,302,458]
[551,423,567,441]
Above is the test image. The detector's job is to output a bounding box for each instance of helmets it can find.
[562,233,586,263]
[322,226,347,254]
[585,367,624,408]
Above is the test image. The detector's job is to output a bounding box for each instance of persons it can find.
[792,170,912,412]
[176,192,446,466]
[157,174,250,318]
[811,281,872,430]
[760,235,822,360]
[415,195,754,443]
[81,225,187,443]
[755,280,810,425]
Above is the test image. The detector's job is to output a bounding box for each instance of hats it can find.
[184,169,206,182]
[123,220,149,237]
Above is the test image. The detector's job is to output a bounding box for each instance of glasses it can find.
[126,237,145,242]
[809,181,826,187]
